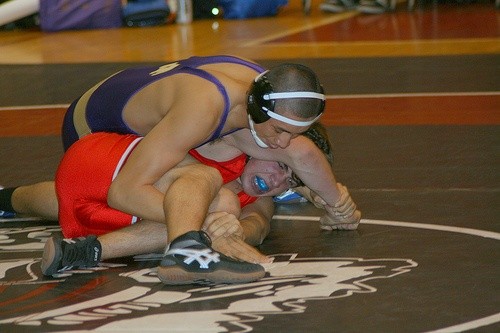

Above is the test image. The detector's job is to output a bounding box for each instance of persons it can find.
[0,53,355,265]
[37,126,362,288]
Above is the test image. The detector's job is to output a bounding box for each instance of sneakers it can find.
[158,231,265,284]
[40,233,103,275]
[273,189,308,202]
